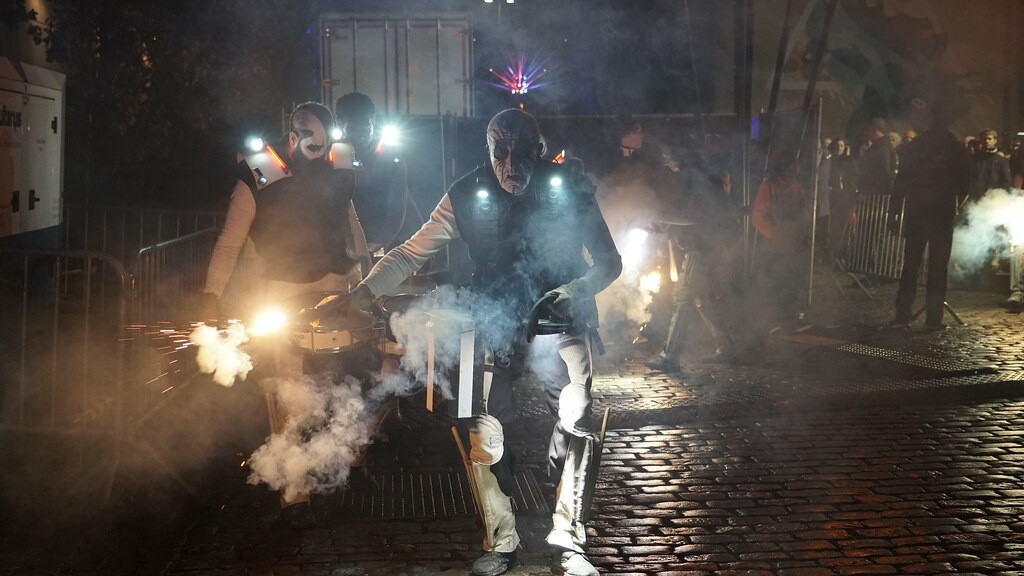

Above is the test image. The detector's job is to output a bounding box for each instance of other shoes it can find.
[472,552,517,576]
[644,356,681,373]
[1006,291,1024,307]
[281,503,317,529]
[889,319,908,328]
[923,322,947,329]
[702,352,737,364]
[549,549,601,576]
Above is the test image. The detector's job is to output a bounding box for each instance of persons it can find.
[318,108,623,576]
[619,97,1024,370]
[200,102,385,510]
[335,93,423,255]
